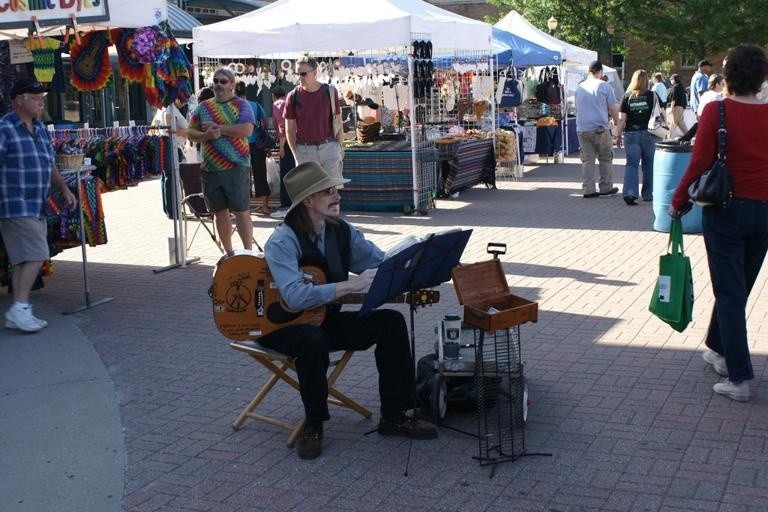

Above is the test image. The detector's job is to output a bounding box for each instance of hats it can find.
[357,116,380,143]
[589,61,602,71]
[10,78,52,100]
[697,59,712,67]
[283,161,351,218]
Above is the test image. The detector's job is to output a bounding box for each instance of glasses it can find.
[326,188,336,195]
[18,95,44,101]
[294,71,307,77]
[213,78,228,84]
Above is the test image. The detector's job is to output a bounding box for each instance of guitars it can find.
[208,248,440,342]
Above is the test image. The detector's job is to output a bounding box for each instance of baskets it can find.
[55,153,85,169]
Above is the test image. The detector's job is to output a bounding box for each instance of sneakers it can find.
[713,377,750,402]
[702,351,727,376]
[5,315,48,329]
[270,209,288,217]
[5,305,42,332]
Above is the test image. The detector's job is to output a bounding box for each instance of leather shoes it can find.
[298,428,324,460]
[583,192,600,198]
[377,419,438,438]
[624,196,638,205]
[599,187,618,194]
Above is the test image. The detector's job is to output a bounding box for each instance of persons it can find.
[150,79,295,219]
[667,46,767,400]
[281,57,348,198]
[181,66,256,270]
[648,58,768,138]
[575,59,622,198]
[252,160,439,460]
[615,67,658,204]
[0,74,78,332]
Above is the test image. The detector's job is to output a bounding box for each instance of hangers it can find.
[498,58,525,77]
[46,123,165,145]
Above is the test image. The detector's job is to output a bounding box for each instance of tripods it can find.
[363,279,486,477]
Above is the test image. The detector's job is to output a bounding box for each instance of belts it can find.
[296,137,334,145]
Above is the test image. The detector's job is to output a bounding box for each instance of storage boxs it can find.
[450,257,539,333]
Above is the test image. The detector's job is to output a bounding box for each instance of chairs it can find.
[180,189,263,259]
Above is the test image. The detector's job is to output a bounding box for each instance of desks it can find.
[337,137,495,215]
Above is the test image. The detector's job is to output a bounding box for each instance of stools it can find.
[230,322,372,444]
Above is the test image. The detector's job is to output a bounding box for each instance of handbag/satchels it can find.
[649,216,694,333]
[647,92,672,139]
[416,354,505,414]
[255,103,275,152]
[536,66,564,105]
[688,102,734,209]
[495,65,536,107]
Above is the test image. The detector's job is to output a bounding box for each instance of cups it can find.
[443,313,462,360]
